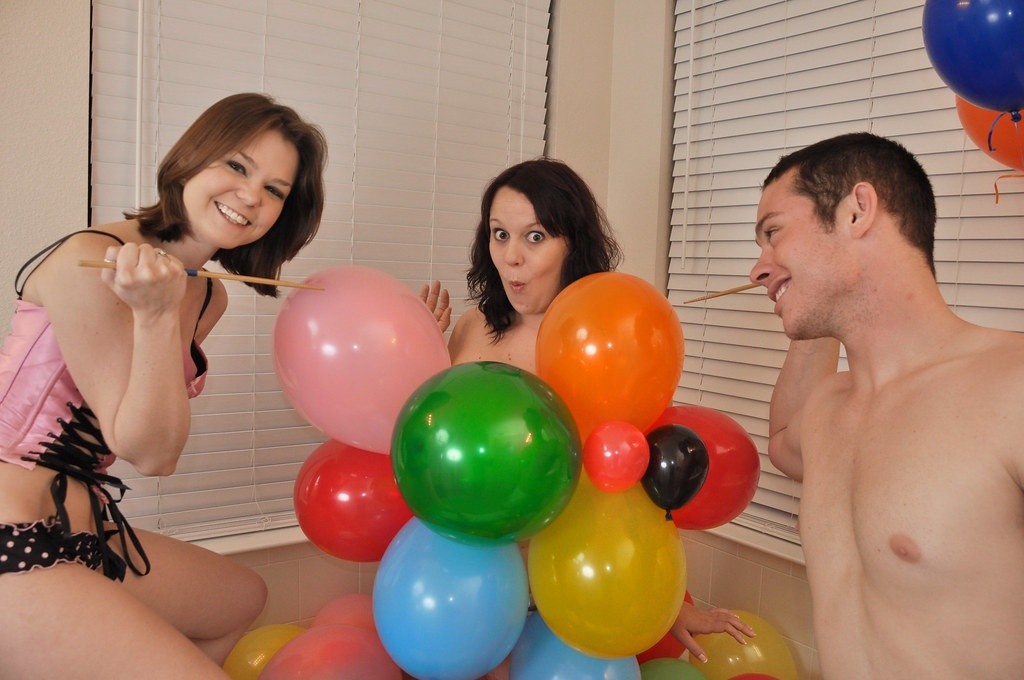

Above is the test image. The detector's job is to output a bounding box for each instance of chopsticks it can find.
[77,261,328,292]
[681,283,760,304]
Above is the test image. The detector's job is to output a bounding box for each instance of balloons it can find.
[217,264,798,680]
[922,0,1024,173]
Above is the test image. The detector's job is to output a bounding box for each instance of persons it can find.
[749,131,1024,680]
[0,94,329,680]
[419,159,757,664]
